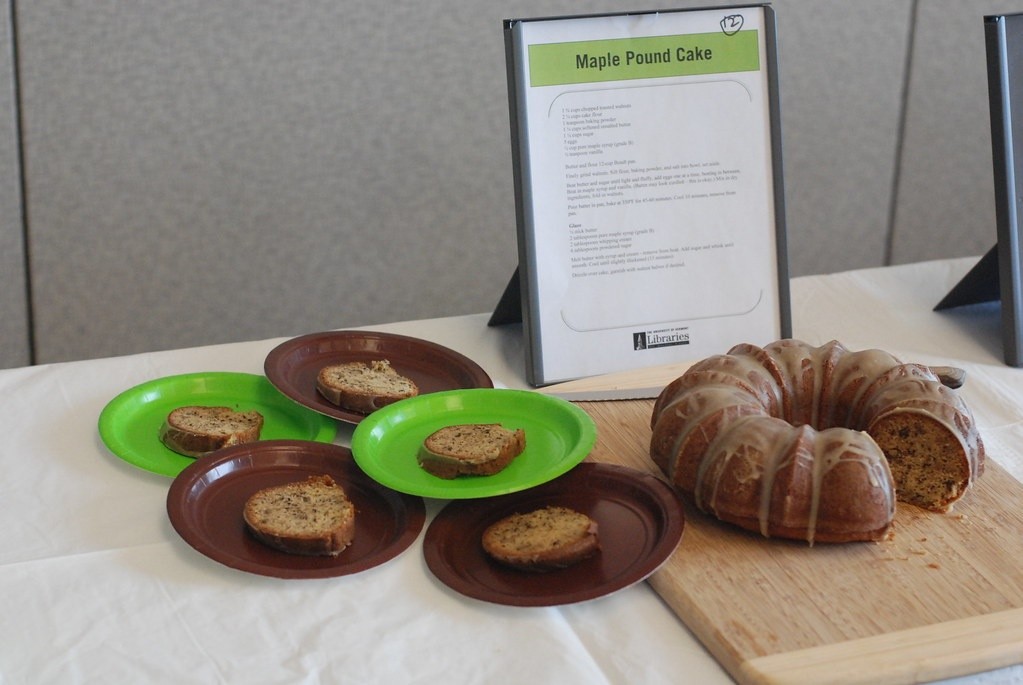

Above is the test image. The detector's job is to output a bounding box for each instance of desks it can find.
[0,255,1022,685]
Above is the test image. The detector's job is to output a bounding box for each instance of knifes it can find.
[540,366,967,402]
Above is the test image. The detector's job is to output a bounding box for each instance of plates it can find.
[166,439,428,580]
[97,371,341,479]
[422,461,687,607]
[351,388,598,501]
[261,329,494,427]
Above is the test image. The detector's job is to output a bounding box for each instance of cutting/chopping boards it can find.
[526,359,1022,685]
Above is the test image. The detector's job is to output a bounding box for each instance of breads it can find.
[242,474,357,555]
[418,422,526,478]
[649,338,985,543]
[316,359,418,414]
[159,405,264,458]
[481,503,599,572]
